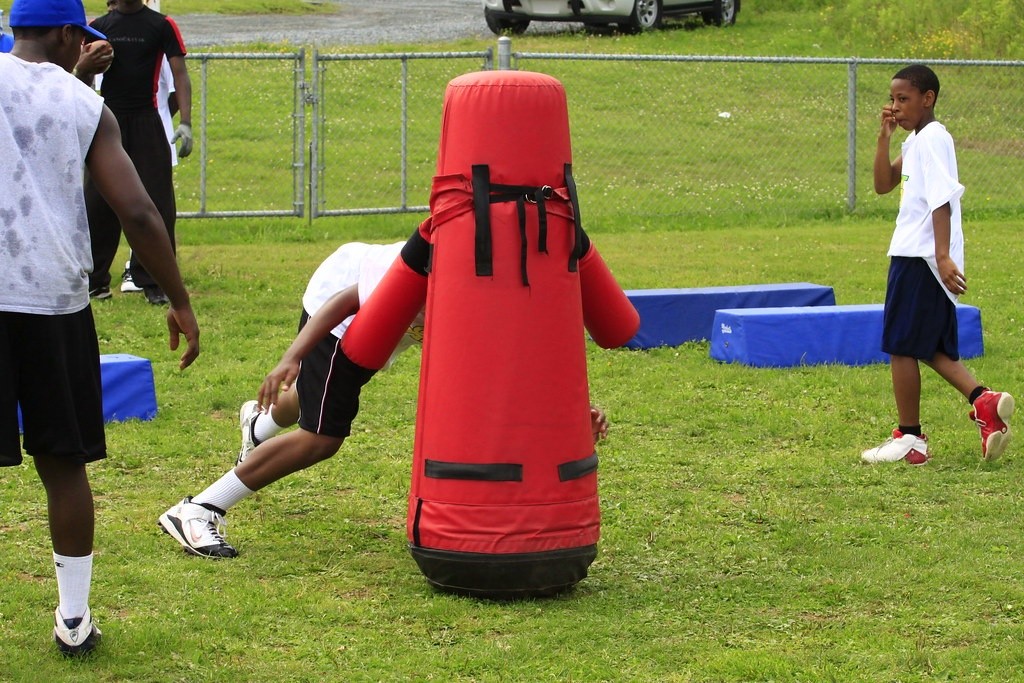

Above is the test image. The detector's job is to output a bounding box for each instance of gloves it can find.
[172,121,192,157]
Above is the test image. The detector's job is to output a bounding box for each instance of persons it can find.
[66,0,194,303]
[94,0,185,293]
[0,0,201,661]
[156,237,432,560]
[856,61,1016,470]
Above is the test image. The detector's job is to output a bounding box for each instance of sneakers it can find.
[236,400,268,468]
[860,429,928,466]
[121,261,144,292]
[144,288,169,304]
[158,496,237,560]
[89,280,112,299]
[969,387,1014,461]
[56,606,100,661]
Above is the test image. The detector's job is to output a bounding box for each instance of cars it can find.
[484,0,742,35]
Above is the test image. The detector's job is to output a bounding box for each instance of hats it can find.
[9,0,107,40]
